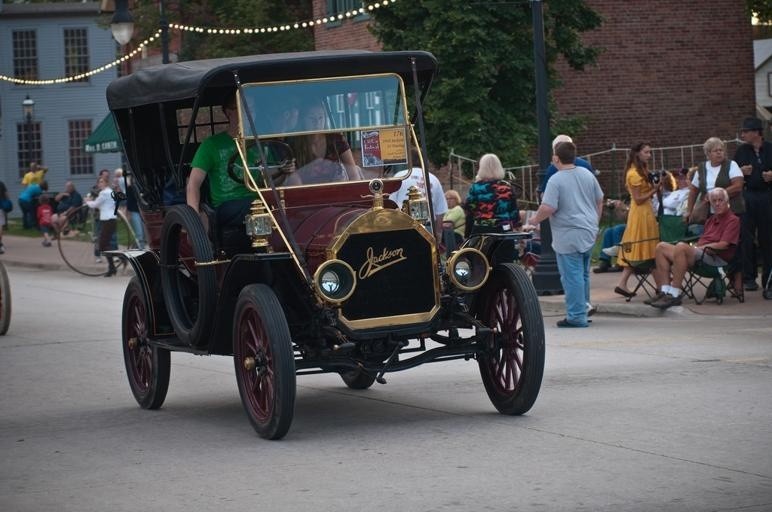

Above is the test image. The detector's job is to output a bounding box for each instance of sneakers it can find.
[593,264,624,274]
[725,279,772,300]
[643,290,683,309]
[0,223,118,278]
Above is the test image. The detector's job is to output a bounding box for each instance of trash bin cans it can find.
[32,191,58,229]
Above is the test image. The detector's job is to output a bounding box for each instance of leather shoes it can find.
[614,286,637,297]
[556,301,597,329]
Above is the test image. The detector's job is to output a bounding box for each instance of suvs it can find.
[95,44,548,438]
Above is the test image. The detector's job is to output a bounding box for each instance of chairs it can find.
[614,226,743,305]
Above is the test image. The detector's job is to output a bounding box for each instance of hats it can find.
[738,116,763,134]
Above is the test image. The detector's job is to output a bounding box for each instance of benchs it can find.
[175,163,250,261]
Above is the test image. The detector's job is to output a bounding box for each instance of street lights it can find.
[23,94,36,165]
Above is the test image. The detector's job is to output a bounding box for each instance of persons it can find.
[186,89,362,237]
[0,181,14,253]
[516,134,604,325]
[18,162,145,278]
[388,144,523,252]
[593,117,772,309]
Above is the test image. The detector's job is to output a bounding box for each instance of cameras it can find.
[648,170,666,184]
